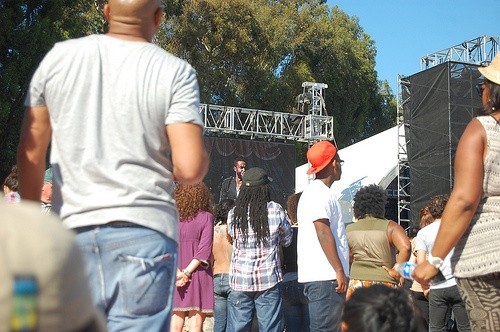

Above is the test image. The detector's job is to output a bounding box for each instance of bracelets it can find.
[395,264,402,272]
[181,269,191,278]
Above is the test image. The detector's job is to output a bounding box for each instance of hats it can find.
[477,51,500,85]
[306,141,337,174]
[1,200,107,331]
[242,167,272,187]
[44,168,54,184]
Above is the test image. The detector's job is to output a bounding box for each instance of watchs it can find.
[427,254,444,270]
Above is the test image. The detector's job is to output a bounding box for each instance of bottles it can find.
[394,262,417,280]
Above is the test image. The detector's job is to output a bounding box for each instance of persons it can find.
[409,50,500,331]
[279,192,314,332]
[230,166,295,332]
[165,178,216,332]
[39,168,54,213]
[209,198,236,332]
[341,183,413,331]
[219,158,248,204]
[296,141,352,332]
[401,193,471,331]
[339,285,417,332]
[16,1,211,332]
[3,165,20,205]
